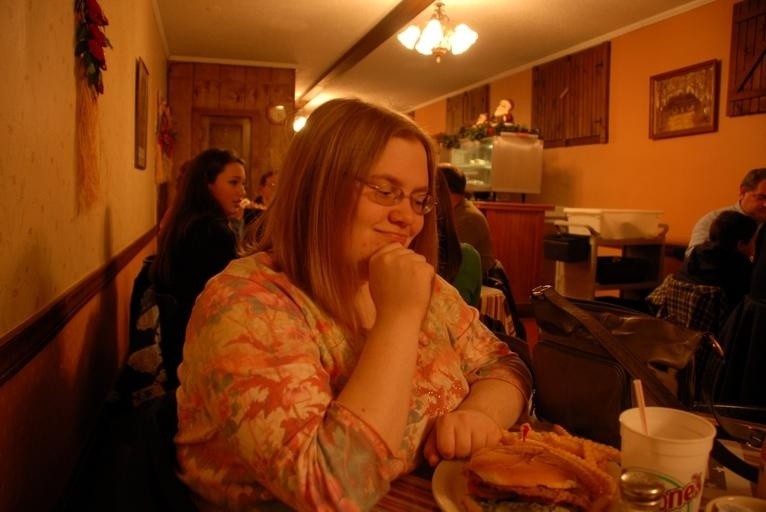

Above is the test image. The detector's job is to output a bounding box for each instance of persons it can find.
[151,148,248,386]
[433,167,483,310]
[484,96,518,138]
[174,97,533,511]
[438,166,497,274]
[685,166,766,265]
[243,171,281,228]
[684,210,756,313]
[156,157,197,245]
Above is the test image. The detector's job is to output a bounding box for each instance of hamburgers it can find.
[460,443,593,511]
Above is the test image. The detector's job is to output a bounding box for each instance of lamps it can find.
[397,2,479,65]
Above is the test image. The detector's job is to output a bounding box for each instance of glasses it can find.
[346,164,438,215]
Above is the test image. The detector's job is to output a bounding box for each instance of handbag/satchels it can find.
[530,284,705,451]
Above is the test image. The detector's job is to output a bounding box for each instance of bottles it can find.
[620,470,666,512]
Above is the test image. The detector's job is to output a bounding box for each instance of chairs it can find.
[662,272,765,380]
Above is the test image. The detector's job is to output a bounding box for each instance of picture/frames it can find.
[646,57,722,141]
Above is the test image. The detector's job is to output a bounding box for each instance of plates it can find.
[430,431,621,512]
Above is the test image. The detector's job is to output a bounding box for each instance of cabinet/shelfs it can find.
[450,131,546,201]
[552,223,668,313]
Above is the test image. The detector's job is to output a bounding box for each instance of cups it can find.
[618,406,718,512]
